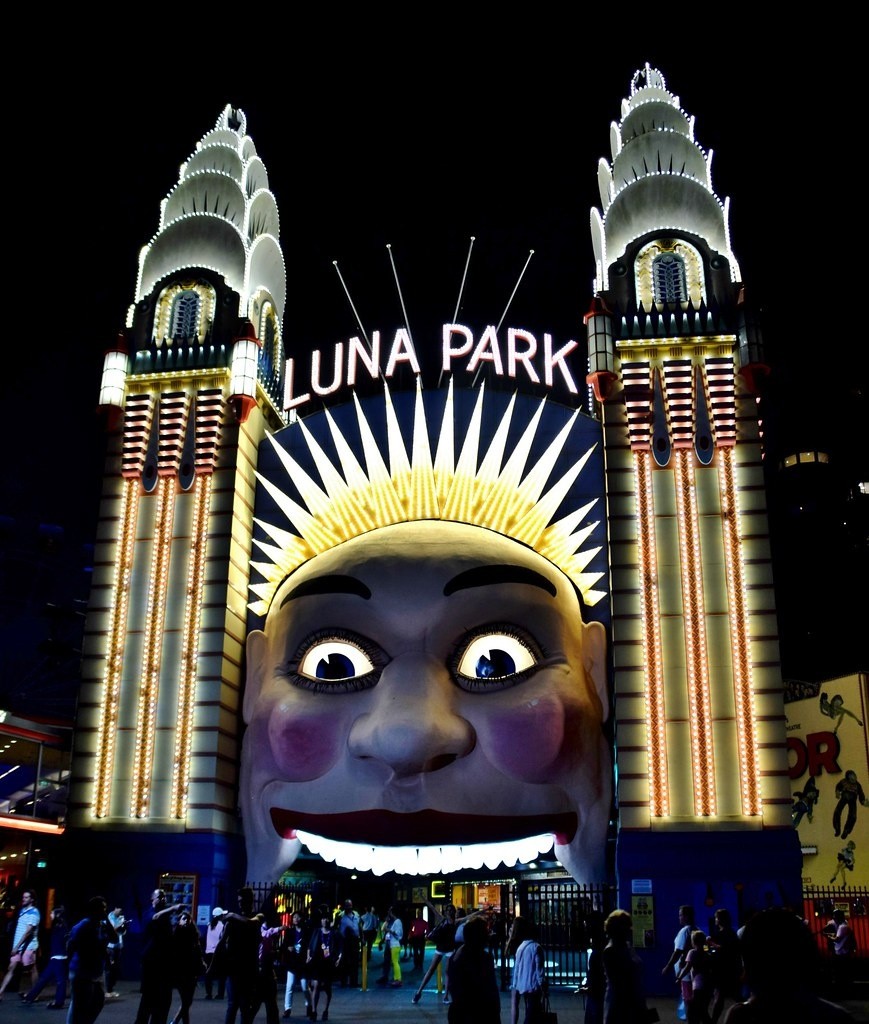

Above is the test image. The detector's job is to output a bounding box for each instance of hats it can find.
[212,907,228,915]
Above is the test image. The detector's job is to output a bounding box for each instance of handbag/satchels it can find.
[427,917,446,942]
[523,1007,558,1024]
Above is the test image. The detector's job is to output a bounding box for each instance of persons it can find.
[602,908,648,1024]
[206,907,230,999]
[662,903,705,1017]
[104,903,127,998]
[207,889,261,1024]
[171,912,207,1024]
[675,929,722,1024]
[513,917,551,1024]
[723,910,859,1024]
[447,916,501,1024]
[711,907,743,1024]
[573,911,608,1024]
[247,910,288,1024]
[795,910,856,1001]
[306,913,344,1022]
[17,905,71,1009]
[63,895,120,1024]
[131,889,190,1024]
[0,889,41,1003]
[412,888,492,1004]
[504,916,526,1024]
[341,902,430,988]
[278,911,313,1018]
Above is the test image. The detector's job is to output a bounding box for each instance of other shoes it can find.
[392,980,403,988]
[310,1012,317,1020]
[18,992,34,1001]
[283,1010,291,1018]
[47,1002,64,1009]
[206,994,224,1001]
[105,992,120,998]
[443,997,451,1003]
[322,1012,329,1020]
[305,1006,313,1016]
[412,993,422,1003]
